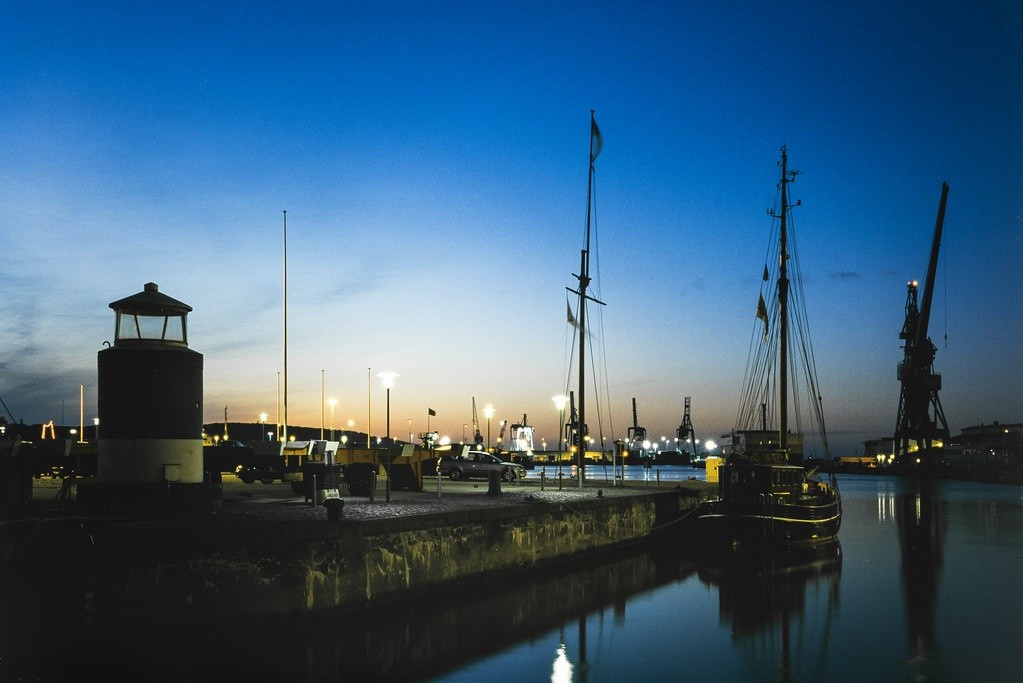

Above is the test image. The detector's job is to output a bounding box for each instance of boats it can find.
[891,446,949,478]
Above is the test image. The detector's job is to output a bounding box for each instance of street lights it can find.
[260,413,267,442]
[329,398,337,441]
[552,394,570,450]
[482,403,494,453]
[378,371,402,501]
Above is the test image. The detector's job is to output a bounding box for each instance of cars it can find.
[436,450,527,482]
[235,454,286,485]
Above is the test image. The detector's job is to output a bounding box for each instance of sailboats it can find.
[686,143,843,547]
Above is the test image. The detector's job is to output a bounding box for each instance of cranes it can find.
[892,182,951,457]
